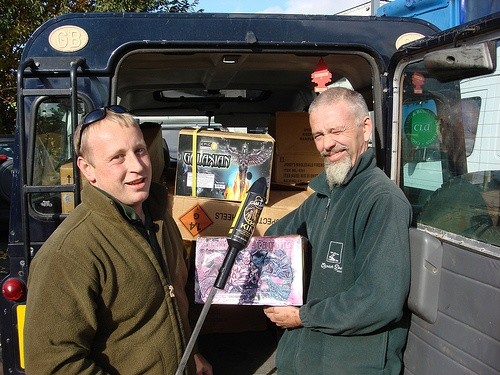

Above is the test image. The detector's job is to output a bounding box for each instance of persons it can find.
[262,87,413,375]
[21,107,213,375]
[411,95,500,249]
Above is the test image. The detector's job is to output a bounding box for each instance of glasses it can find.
[78,105,127,148]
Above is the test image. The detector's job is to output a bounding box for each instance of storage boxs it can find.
[58,110,328,308]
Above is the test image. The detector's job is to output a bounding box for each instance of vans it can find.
[0,8,499,375]
